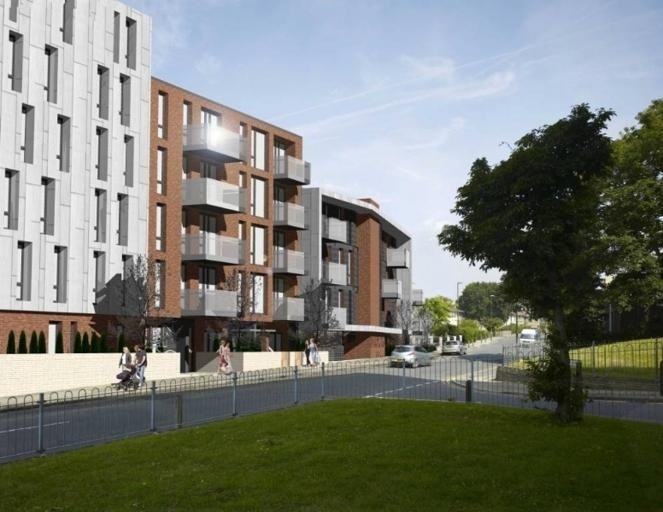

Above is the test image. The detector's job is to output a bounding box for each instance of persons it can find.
[304,338,318,366]
[216,340,231,373]
[133,343,147,387]
[118,347,132,371]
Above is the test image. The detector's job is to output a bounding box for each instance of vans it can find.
[519,328,541,348]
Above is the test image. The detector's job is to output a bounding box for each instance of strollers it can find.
[112,363,140,392]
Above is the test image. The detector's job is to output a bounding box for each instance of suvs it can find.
[390,344,434,367]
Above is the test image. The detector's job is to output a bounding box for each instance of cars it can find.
[443,340,466,355]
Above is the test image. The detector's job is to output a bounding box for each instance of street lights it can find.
[456,281,463,329]
[489,294,494,334]
